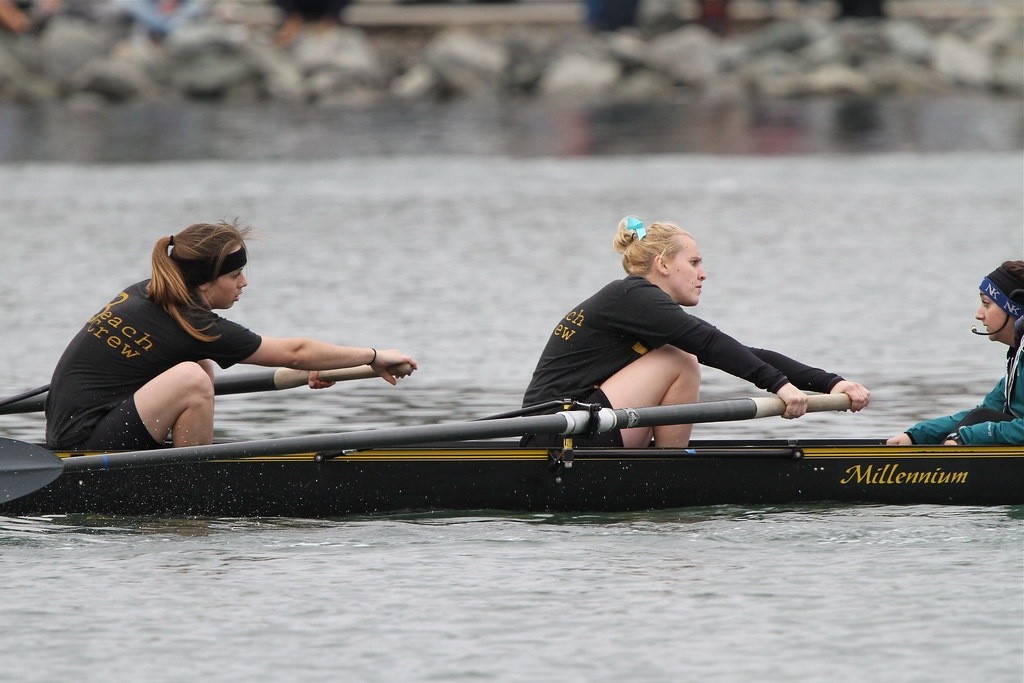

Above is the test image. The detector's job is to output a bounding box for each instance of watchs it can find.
[947,432,963,445]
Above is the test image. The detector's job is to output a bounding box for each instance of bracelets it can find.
[367,348,376,365]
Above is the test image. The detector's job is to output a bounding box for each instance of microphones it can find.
[971,314,1009,335]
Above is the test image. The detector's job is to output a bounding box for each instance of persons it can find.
[0,0,352,49]
[886,261,1024,445]
[45,223,417,450]
[522,216,870,449]
[588,0,886,28]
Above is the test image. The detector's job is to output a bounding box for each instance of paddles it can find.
[0,361,414,413]
[0,393,850,505]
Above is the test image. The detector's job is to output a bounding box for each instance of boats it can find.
[1,438,1023,514]
